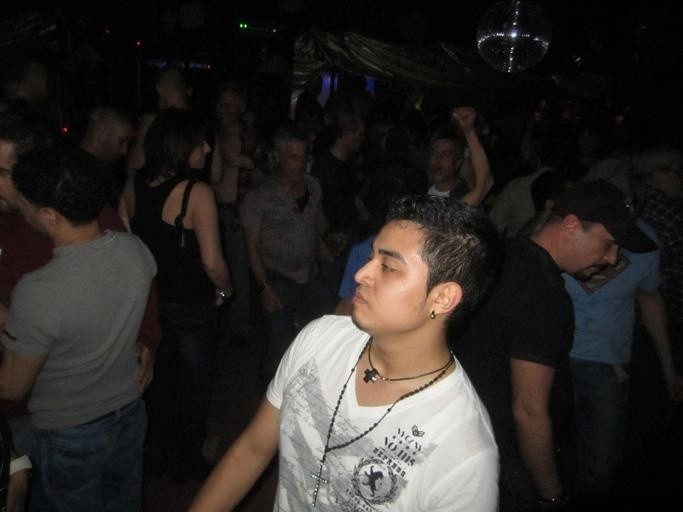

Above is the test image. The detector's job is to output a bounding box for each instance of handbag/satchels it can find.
[169,179,216,305]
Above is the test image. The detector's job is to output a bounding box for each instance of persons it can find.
[0,55,683,511]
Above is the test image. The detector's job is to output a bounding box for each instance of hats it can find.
[552,179,658,253]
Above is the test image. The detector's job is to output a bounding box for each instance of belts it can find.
[78,400,135,428]
